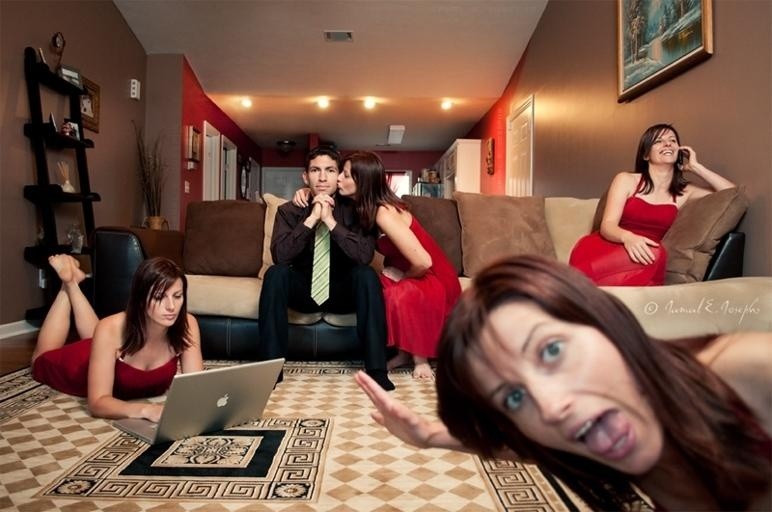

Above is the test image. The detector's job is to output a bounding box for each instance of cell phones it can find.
[678,147,685,164]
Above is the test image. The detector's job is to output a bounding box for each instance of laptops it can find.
[111,356,285,447]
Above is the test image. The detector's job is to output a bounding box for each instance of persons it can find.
[355,255,772,512]
[257,143,396,392]
[292,152,463,380]
[569,124,737,286]
[31,254,204,424]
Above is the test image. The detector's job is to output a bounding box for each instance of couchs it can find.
[91,191,772,361]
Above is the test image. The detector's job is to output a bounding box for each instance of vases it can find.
[145,216,166,230]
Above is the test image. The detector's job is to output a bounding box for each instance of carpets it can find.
[0,360,655,512]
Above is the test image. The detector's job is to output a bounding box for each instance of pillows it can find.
[401,194,462,276]
[258,193,289,280]
[452,192,556,278]
[183,199,263,276]
[659,184,756,286]
[543,197,600,266]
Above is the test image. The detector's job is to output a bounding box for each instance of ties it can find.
[310,220,331,308]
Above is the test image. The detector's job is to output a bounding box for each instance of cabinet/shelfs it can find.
[23,47,102,328]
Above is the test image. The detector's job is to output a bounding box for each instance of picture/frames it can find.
[80,75,100,133]
[617,0,713,103]
[189,126,202,162]
